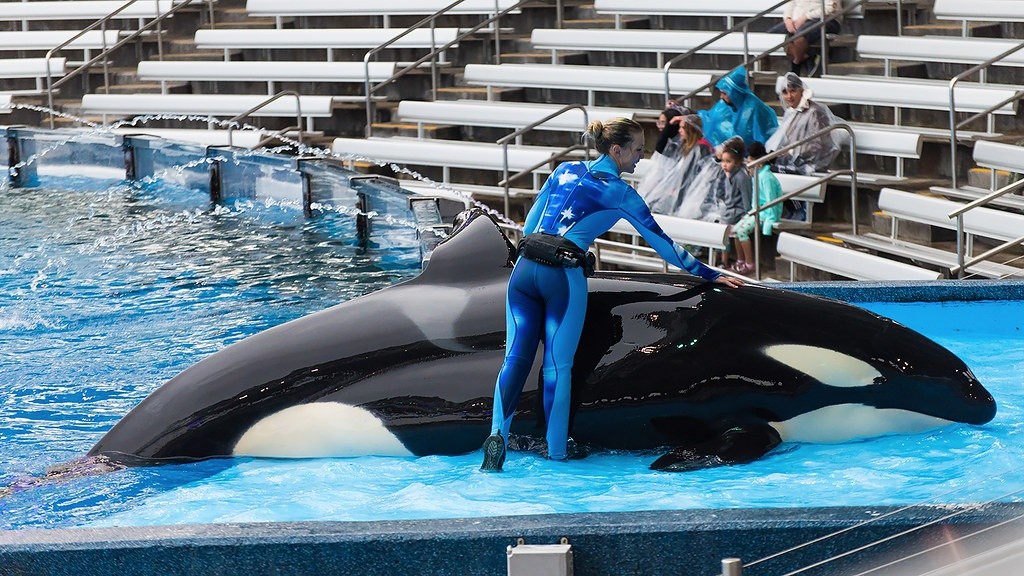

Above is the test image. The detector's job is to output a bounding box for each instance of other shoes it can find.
[790,208,806,221]
[730,262,753,274]
[781,206,791,219]
[804,53,821,78]
[791,61,803,77]
[478,433,506,473]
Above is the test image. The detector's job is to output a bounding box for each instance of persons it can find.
[480,118,744,473]
[782,0,842,77]
[632,63,842,276]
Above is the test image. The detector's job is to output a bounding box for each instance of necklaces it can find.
[587,160,619,180]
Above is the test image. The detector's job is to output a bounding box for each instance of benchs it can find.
[0,0,1024,281]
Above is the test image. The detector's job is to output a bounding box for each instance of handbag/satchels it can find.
[515,233,596,278]
